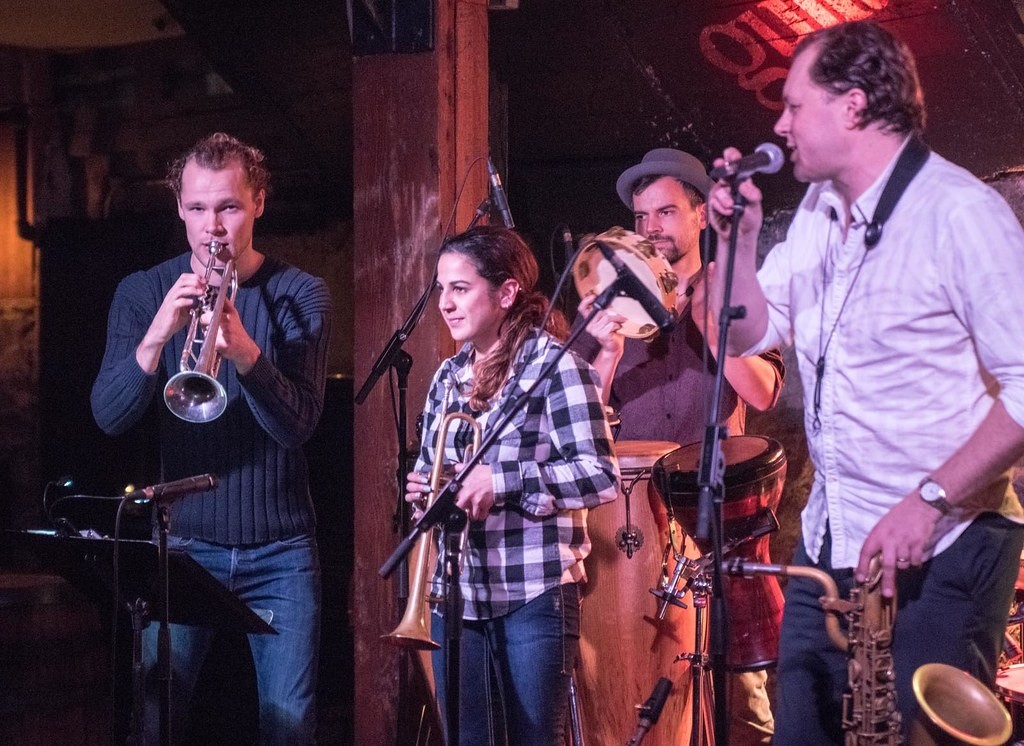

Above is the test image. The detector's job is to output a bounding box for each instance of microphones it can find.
[487,158,516,229]
[1002,634,1022,661]
[708,142,784,179]
[597,239,677,332]
[562,228,576,274]
[124,472,219,504]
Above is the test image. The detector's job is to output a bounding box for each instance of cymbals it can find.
[570,225,680,344]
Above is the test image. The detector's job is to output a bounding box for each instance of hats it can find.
[612,148,718,214]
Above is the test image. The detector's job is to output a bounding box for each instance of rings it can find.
[897,557,910,564]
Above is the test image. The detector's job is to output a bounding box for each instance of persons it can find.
[700,18,1024,745]
[556,148,789,746]
[398,223,622,746]
[90,130,336,745]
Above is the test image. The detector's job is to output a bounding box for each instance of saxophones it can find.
[721,549,1013,746]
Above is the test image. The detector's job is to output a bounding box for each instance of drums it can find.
[651,435,789,675]
[570,439,708,746]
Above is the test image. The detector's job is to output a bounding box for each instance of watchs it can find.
[918,476,953,515]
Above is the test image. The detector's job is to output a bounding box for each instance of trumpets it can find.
[379,379,482,651]
[163,241,238,425]
